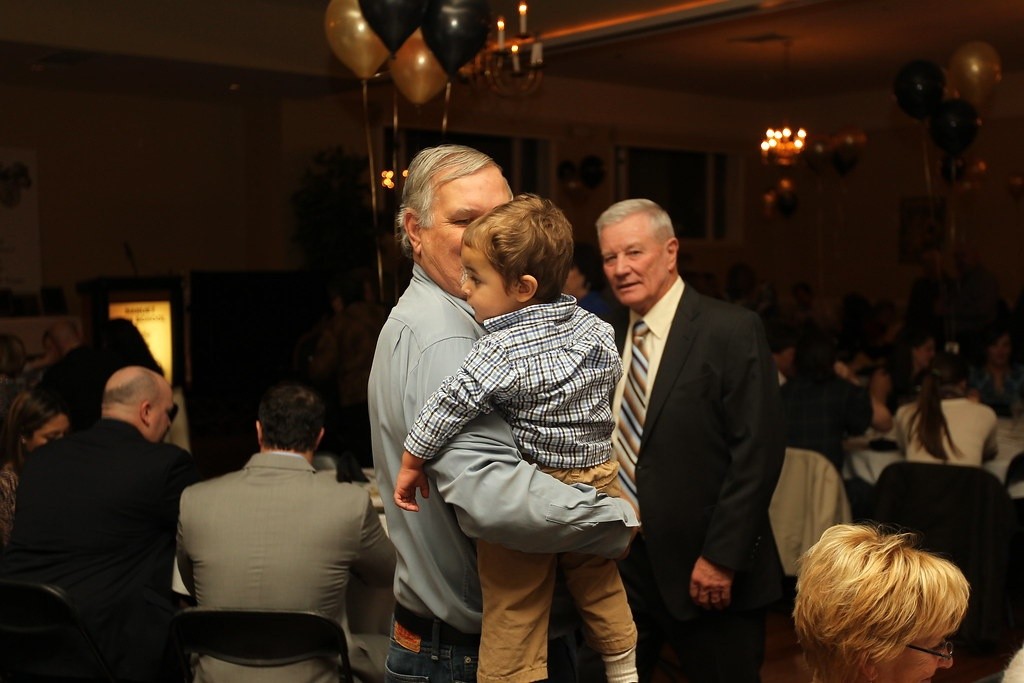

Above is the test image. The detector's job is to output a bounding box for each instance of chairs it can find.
[167,610,353,683]
[0,577,110,683]
[865,461,1017,655]
[768,448,854,583]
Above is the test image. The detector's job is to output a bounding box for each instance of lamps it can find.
[759,116,808,171]
[475,0,545,96]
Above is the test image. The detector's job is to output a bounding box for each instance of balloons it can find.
[830,146,858,176]
[942,39,1002,105]
[557,154,605,189]
[929,98,979,186]
[894,58,944,123]
[1007,171,1024,202]
[778,190,799,219]
[324,0,449,108]
[360,0,490,87]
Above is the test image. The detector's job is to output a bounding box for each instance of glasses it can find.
[907,642,953,663]
[152,402,178,425]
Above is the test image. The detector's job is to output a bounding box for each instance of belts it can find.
[393,602,587,645]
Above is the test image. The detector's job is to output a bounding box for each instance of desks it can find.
[843,418,1023,510]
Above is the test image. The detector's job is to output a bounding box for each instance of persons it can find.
[0,372,70,551]
[682,241,1023,480]
[308,232,415,458]
[594,197,788,683]
[0,364,204,683]
[0,319,164,468]
[369,145,641,683]
[393,194,639,683]
[792,520,971,683]
[560,240,617,322]
[175,385,397,683]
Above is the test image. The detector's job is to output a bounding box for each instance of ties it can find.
[616,322,651,496]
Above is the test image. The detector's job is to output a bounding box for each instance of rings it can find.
[690,581,699,588]
